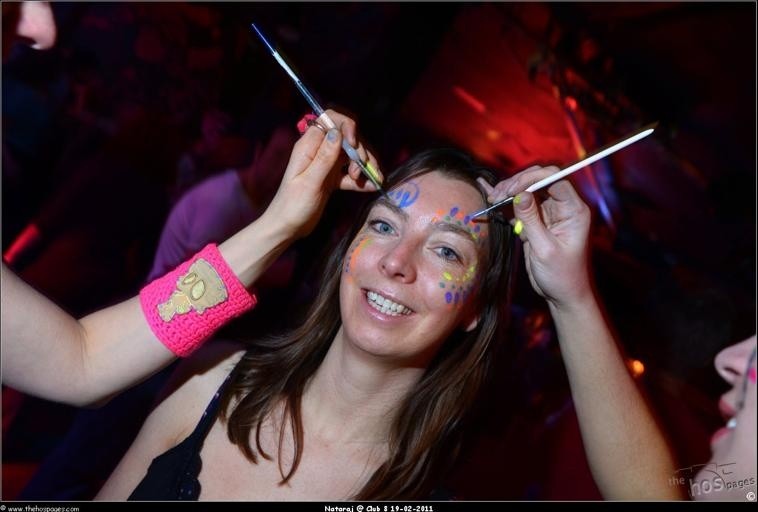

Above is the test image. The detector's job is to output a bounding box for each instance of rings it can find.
[293,115,324,136]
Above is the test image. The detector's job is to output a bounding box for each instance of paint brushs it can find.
[464,126,654,226]
[235,4,389,205]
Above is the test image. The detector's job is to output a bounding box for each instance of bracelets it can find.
[136,241,259,364]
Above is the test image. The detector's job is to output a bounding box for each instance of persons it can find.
[0,107,384,412]
[469,163,756,502]
[145,126,297,278]
[91,142,530,500]
